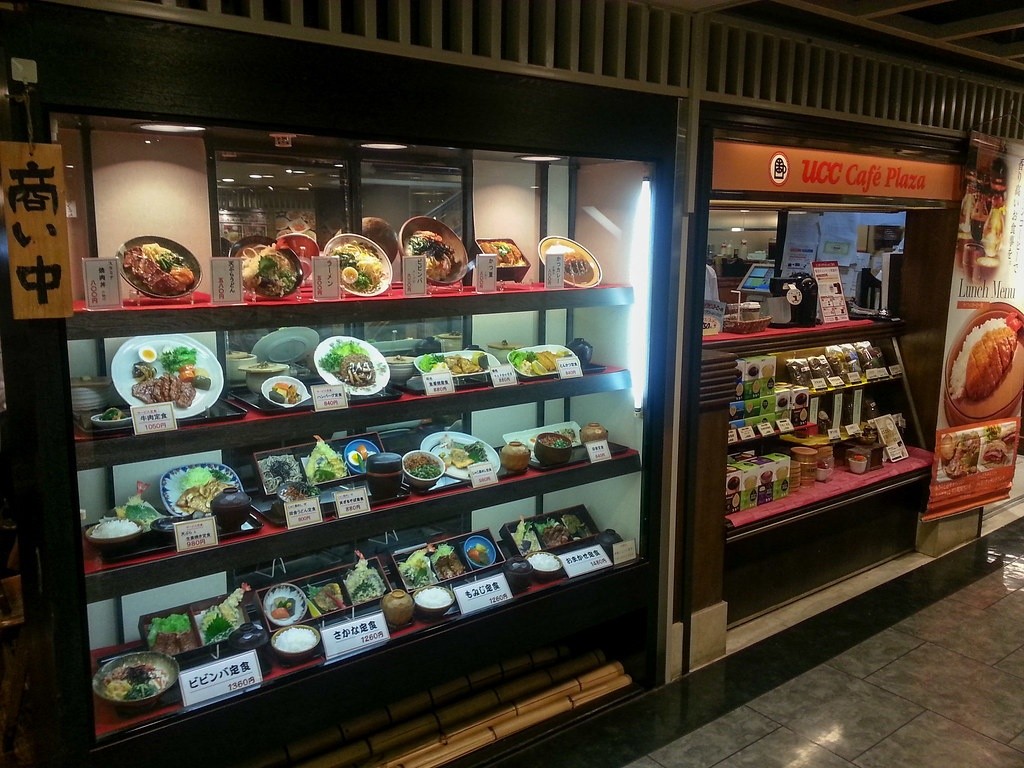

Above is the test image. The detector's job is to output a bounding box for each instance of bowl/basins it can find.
[226,354,258,382]
[384,355,415,386]
[500,423,608,473]
[85,450,446,548]
[416,332,463,356]
[462,337,593,368]
[92,528,623,739]
[238,363,312,408]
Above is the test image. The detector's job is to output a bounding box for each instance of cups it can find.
[816,455,868,481]
[741,296,791,324]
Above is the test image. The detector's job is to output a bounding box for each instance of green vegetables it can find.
[157,253,184,270]
[419,353,445,371]
[178,468,232,488]
[508,349,538,368]
[254,255,296,297]
[146,611,234,647]
[408,439,490,481]
[550,440,567,448]
[524,518,557,537]
[159,346,198,372]
[122,683,156,700]
[335,251,372,291]
[318,341,368,374]
[408,234,433,255]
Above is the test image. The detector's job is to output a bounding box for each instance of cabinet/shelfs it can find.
[65,281,648,768]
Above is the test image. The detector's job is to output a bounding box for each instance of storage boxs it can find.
[728,355,810,427]
[726,451,790,515]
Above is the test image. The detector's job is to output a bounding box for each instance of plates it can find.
[220,217,398,280]
[137,504,621,657]
[251,327,423,365]
[116,216,601,298]
[420,421,583,481]
[314,335,390,395]
[226,418,467,469]
[91,334,224,428]
[160,431,385,516]
[415,343,581,378]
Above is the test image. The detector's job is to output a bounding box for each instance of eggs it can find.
[342,267,359,283]
[348,450,364,465]
[138,345,157,363]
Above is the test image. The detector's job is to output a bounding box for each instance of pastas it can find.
[328,240,386,293]
[403,230,451,280]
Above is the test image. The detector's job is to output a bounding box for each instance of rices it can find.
[950,317,1007,399]
[274,628,317,653]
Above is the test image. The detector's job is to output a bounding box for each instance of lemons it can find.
[532,360,549,374]
